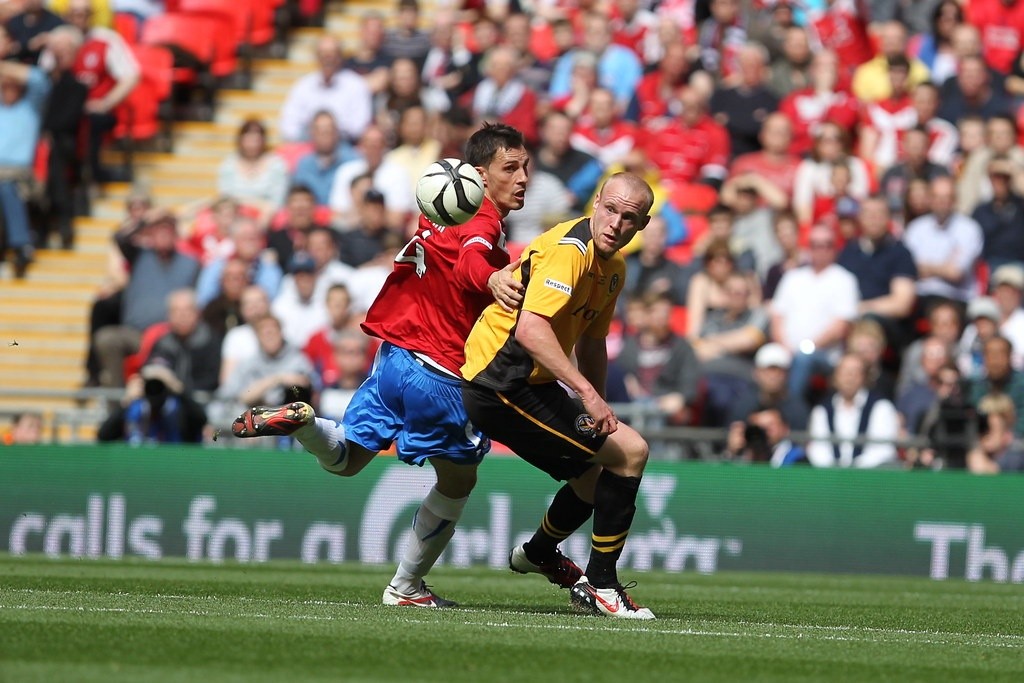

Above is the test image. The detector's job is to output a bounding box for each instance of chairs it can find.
[94,0,320,184]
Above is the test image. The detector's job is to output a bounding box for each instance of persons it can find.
[232,120,529,606]
[76,0,1024,469]
[13,413,44,442]
[461,172,656,619]
[0,0,143,278]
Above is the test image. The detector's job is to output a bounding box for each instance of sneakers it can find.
[570,574,655,621]
[508,542,585,589]
[383,581,459,610]
[230,401,315,439]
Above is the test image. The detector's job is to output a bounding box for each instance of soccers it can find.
[415,157,485,225]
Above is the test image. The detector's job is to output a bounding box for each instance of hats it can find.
[754,344,793,371]
[967,295,1001,322]
[990,262,1024,291]
